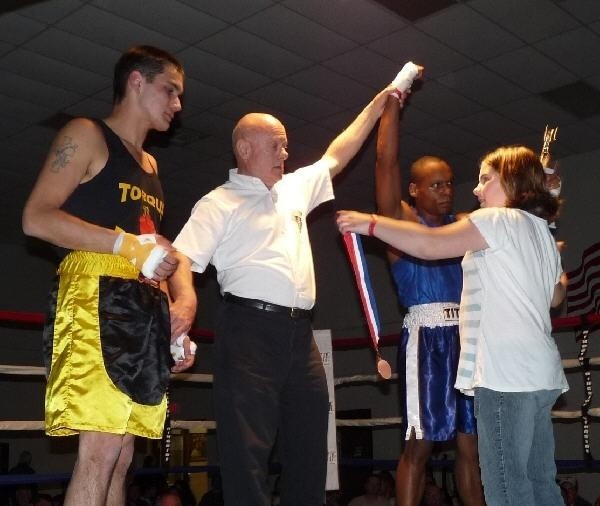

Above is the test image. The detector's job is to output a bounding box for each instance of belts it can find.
[230,296,313,323]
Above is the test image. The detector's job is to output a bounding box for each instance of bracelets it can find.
[368,213,378,237]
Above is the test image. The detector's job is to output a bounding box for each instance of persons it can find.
[168,84,412,506]
[375,60,562,506]
[335,144,568,505]
[0,461,589,506]
[21,41,197,505]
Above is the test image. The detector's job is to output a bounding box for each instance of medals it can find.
[377,357,393,381]
